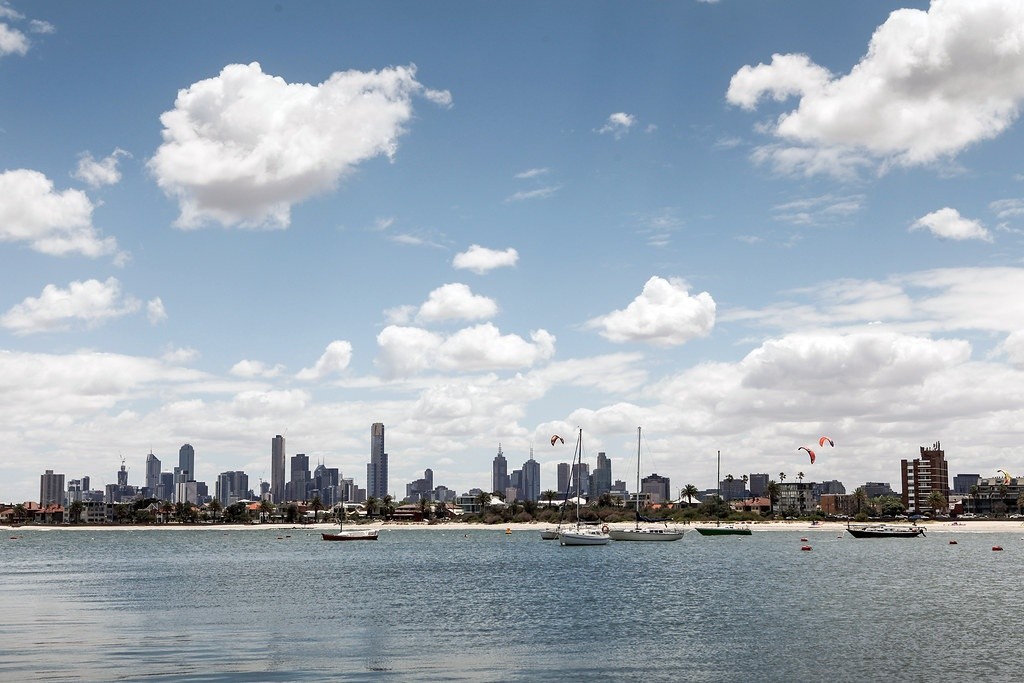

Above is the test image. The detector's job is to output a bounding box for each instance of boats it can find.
[808,525,821,529]
[844,516,929,538]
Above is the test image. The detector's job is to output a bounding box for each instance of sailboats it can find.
[536,428,580,540]
[556,426,612,545]
[320,489,380,540]
[610,426,684,541]
[693,450,754,535]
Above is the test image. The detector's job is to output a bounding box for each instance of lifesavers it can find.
[602,526,609,534]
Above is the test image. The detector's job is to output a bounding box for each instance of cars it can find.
[828,513,855,520]
[894,514,951,520]
[1010,514,1024,518]
[957,513,988,519]
